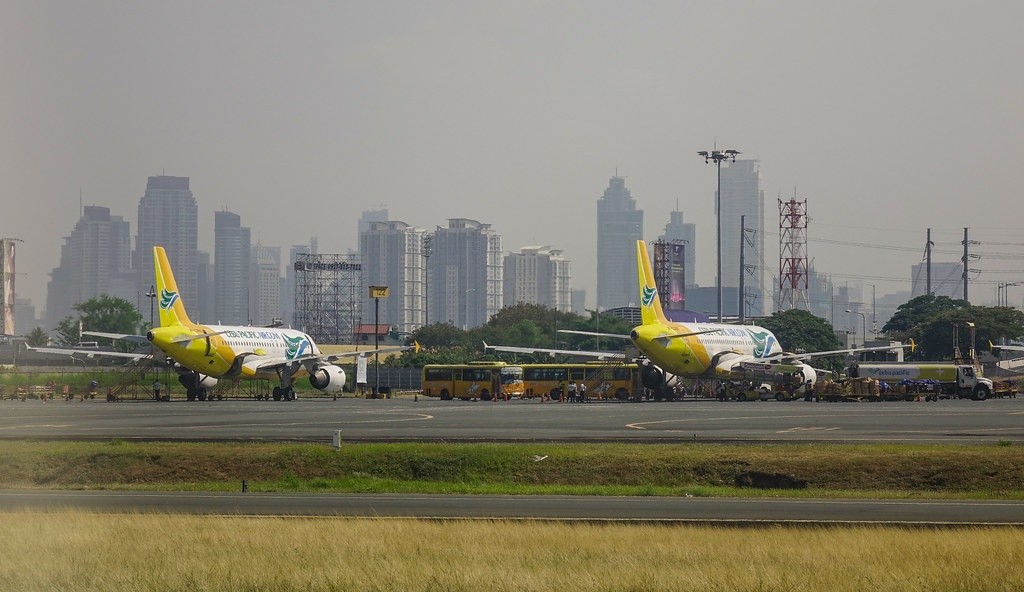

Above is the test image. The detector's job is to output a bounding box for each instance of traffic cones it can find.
[596,392,603,402]
[545,392,553,401]
[539,391,545,402]
[558,392,565,403]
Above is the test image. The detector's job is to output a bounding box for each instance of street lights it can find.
[697,150,743,323]
[846,310,865,348]
[421,234,432,326]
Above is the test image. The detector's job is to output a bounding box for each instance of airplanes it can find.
[23,242,420,402]
[482,241,919,402]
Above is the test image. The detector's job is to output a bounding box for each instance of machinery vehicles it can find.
[846,358,993,402]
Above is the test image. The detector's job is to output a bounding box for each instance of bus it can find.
[419,361,524,401]
[518,360,639,401]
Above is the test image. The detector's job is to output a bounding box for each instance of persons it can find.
[558,380,586,403]
[804,379,813,402]
[153,379,161,402]
[715,381,728,402]
